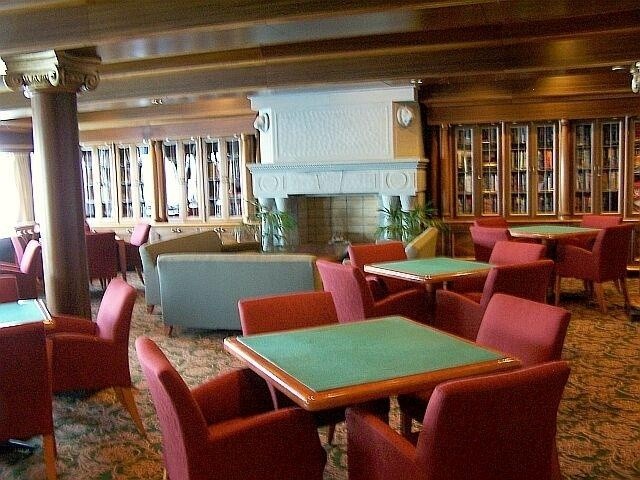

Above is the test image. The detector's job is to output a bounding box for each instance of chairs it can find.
[46,276,151,444]
[2,214,634,344]
[2,322,57,478]
[345,360,570,478]
[238,292,390,443]
[398,294,571,434]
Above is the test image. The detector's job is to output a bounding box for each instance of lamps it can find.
[630,61,640,93]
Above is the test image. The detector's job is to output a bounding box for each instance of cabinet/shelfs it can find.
[426,98,640,272]
[29,114,259,245]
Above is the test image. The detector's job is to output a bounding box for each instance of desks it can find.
[224,314,523,411]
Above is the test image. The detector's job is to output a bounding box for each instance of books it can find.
[80,138,241,218]
[453,126,618,213]
